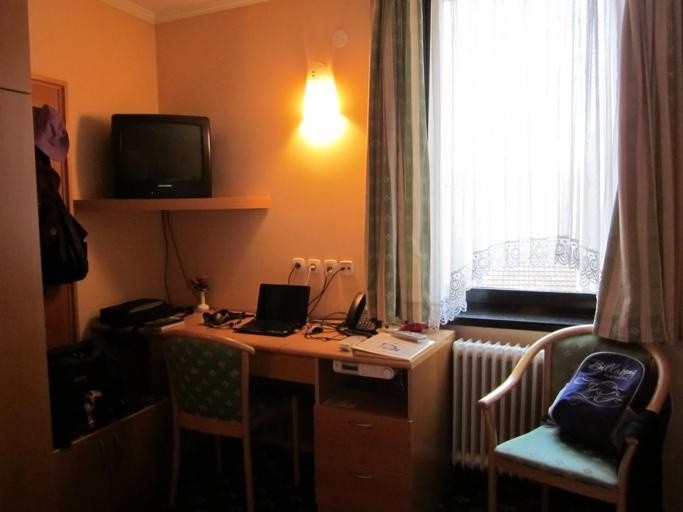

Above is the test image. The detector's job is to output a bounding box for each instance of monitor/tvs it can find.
[111,113,213,200]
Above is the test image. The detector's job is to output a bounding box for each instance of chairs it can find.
[468,320,672,509]
[154,322,303,509]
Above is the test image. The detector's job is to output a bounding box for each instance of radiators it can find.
[445,336,548,485]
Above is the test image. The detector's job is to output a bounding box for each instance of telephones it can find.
[345,292,377,331]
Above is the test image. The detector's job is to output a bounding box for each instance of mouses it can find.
[307,325,324,334]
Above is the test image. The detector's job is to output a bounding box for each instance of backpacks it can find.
[543,348,646,461]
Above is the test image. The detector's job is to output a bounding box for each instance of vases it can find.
[196,291,210,311]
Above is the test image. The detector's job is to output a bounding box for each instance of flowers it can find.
[189,275,209,296]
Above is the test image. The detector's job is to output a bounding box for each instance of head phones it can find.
[203,308,232,326]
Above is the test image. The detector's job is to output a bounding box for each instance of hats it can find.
[32,104,70,162]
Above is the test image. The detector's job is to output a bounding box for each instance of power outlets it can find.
[290,257,354,277]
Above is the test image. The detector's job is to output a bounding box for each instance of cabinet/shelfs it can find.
[52,394,172,512]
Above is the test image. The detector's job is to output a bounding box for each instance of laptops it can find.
[235,283,310,337]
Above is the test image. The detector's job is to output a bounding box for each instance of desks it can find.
[91,298,456,511]
